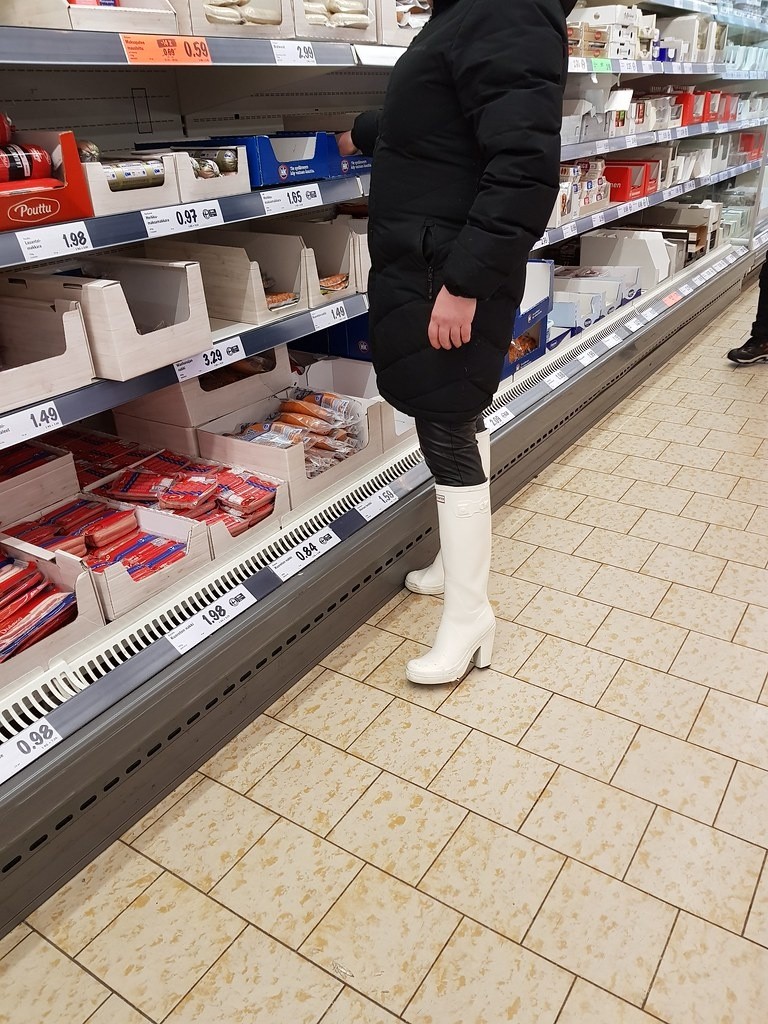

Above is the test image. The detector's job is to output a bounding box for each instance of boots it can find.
[405,428,491,597]
[405,481,497,686]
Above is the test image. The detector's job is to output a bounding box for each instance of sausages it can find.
[0,0,425,660]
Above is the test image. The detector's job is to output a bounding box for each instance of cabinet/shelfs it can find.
[0,0,768,942]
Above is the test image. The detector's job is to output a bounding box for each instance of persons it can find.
[338,0,581,685]
[727,250,767,365]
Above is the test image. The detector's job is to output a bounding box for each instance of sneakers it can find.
[727,337,768,364]
